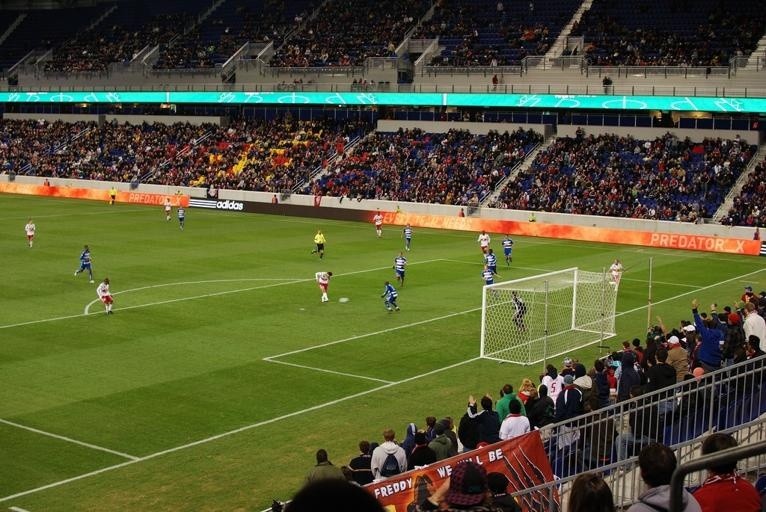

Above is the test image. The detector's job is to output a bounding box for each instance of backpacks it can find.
[379,454,402,478]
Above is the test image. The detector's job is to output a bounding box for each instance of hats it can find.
[682,324,696,332]
[564,374,573,383]
[488,471,509,490]
[744,286,752,291]
[692,367,704,375]
[446,461,485,507]
[727,312,740,321]
[668,336,680,345]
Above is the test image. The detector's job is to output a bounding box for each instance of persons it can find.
[393,251,407,285]
[96,278,114,316]
[312,230,326,261]
[315,272,333,303]
[477,230,491,255]
[25,218,35,249]
[374,212,384,238]
[610,258,623,290]
[75,245,95,284]
[502,234,514,266]
[402,223,413,250]
[482,264,501,294]
[485,248,498,274]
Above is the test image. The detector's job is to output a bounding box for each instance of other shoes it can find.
[312,249,315,253]
[74,270,78,277]
[406,245,410,252]
[386,308,393,311]
[322,296,328,303]
[395,307,400,310]
[107,310,112,314]
[89,280,94,284]
